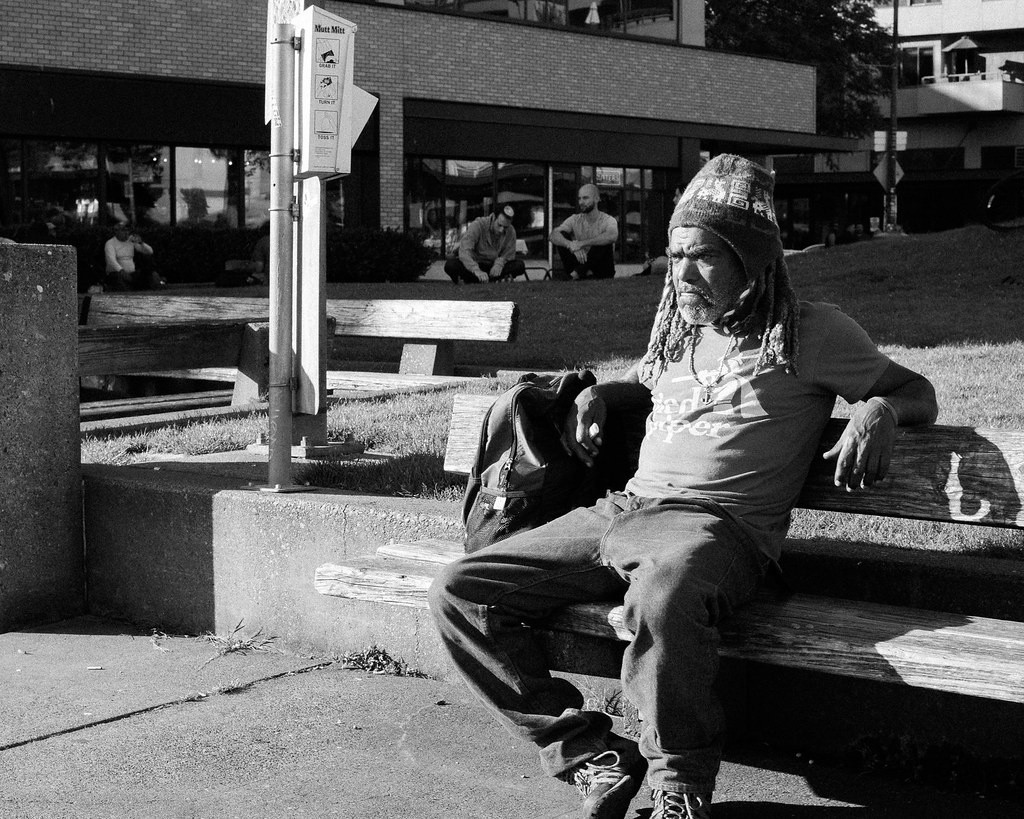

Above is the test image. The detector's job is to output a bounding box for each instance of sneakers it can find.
[650,787,713,818]
[560,735,648,819]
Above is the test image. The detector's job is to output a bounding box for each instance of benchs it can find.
[315,391,1024,707]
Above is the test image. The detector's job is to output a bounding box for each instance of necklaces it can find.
[691,330,734,403]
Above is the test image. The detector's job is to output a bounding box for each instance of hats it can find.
[641,152,802,381]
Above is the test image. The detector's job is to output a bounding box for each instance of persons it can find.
[826,222,870,248]
[104,221,166,290]
[548,184,618,280]
[444,202,524,285]
[428,152,940,819]
[631,185,688,277]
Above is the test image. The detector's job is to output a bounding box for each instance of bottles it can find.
[126,233,143,245]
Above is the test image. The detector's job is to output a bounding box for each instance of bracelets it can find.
[866,394,898,427]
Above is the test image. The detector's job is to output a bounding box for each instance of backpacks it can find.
[463,370,596,552]
